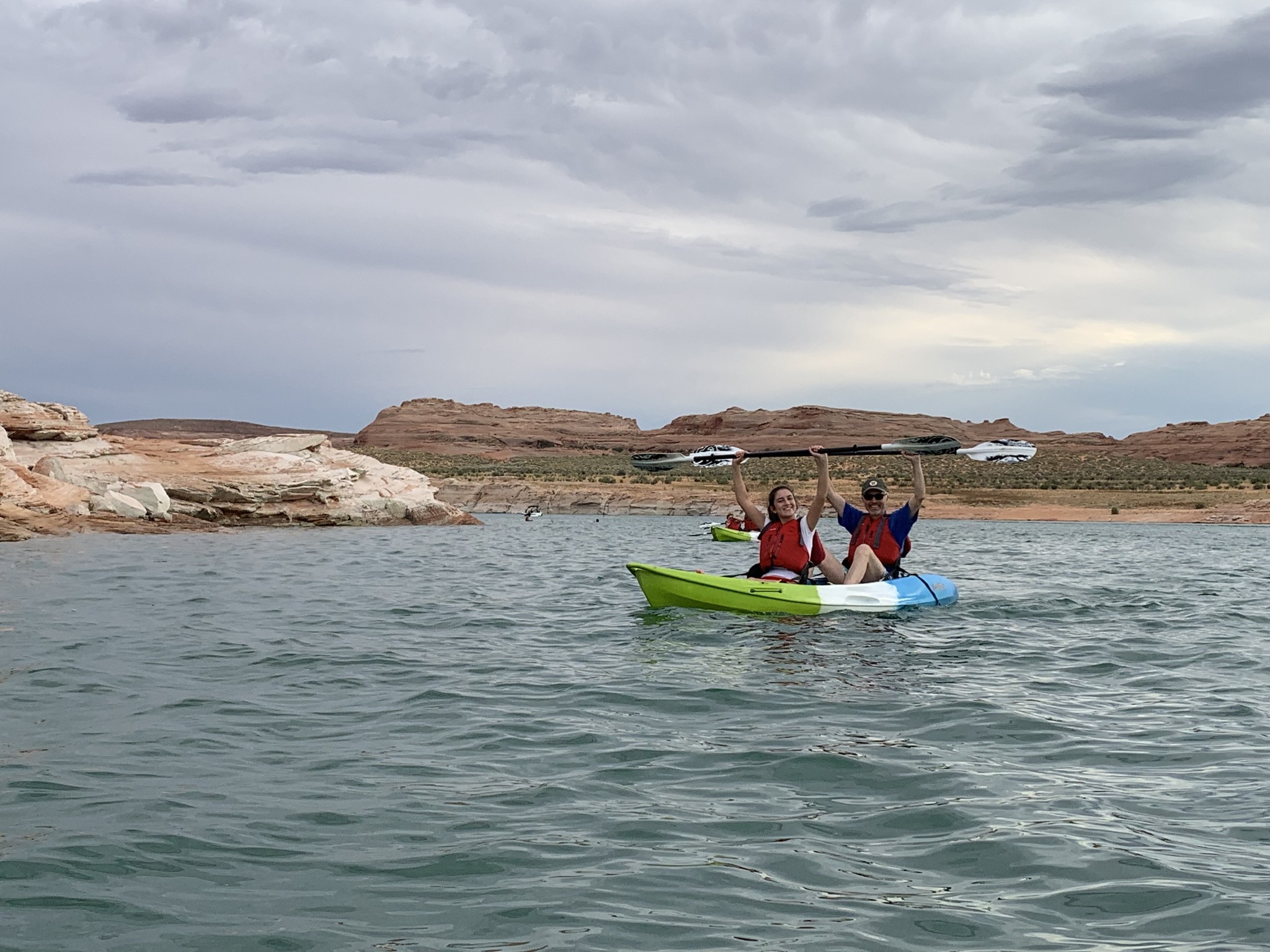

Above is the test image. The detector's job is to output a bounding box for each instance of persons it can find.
[807,450,926,585]
[731,445,829,585]
[726,514,743,531]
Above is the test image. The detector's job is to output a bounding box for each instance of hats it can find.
[861,479,887,494]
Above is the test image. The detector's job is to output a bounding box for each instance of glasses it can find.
[863,493,887,500]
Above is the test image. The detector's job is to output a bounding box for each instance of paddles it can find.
[688,532,711,537]
[697,517,751,528]
[629,435,963,473]
[690,437,1042,463]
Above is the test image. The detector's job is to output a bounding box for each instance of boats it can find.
[521,505,544,517]
[626,562,960,616]
[710,525,761,544]
